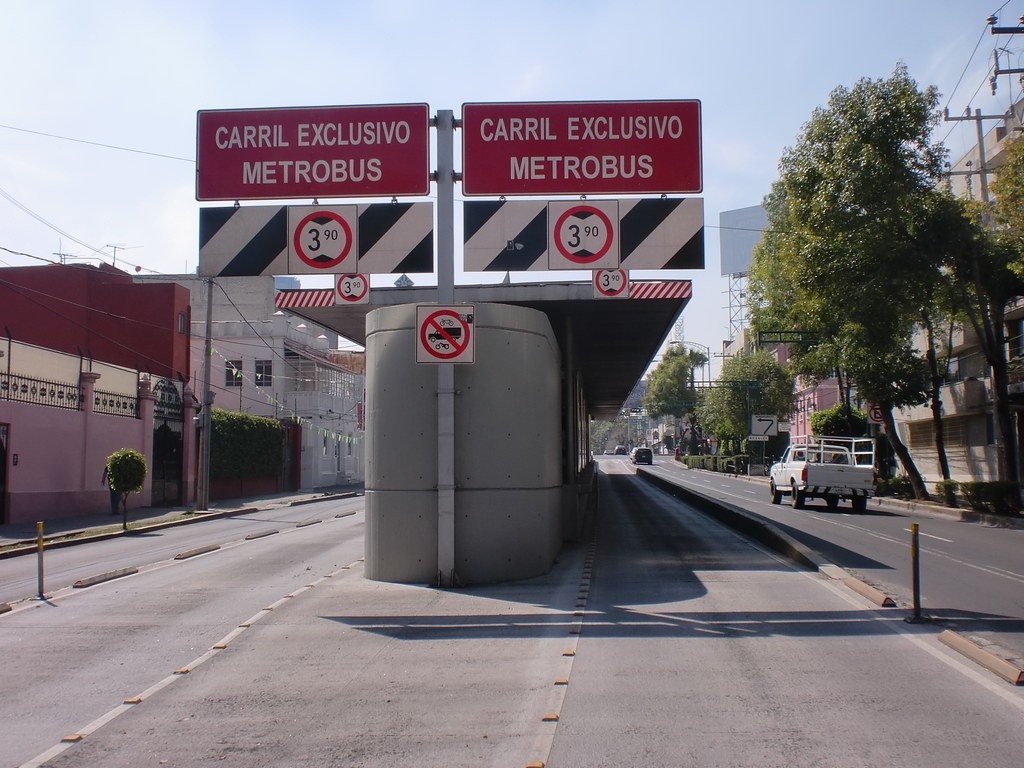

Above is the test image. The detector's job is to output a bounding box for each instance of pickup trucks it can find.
[769,435,877,512]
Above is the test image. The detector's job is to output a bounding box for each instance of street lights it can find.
[668,339,710,390]
[652,360,695,392]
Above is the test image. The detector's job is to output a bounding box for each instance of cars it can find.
[604,450,613,455]
[614,445,627,455]
[630,447,652,465]
